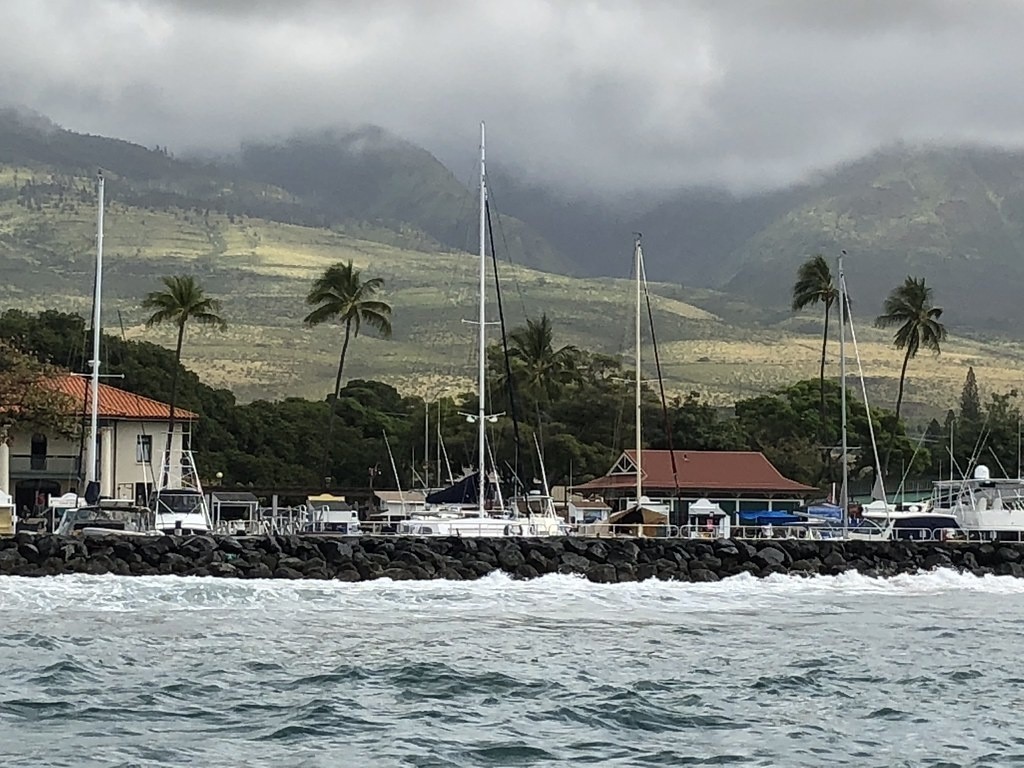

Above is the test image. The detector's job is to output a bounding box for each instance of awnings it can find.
[735,507,798,538]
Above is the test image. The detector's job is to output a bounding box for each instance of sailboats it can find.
[23,120,1024,540]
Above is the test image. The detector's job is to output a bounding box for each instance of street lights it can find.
[216,472,223,487]
